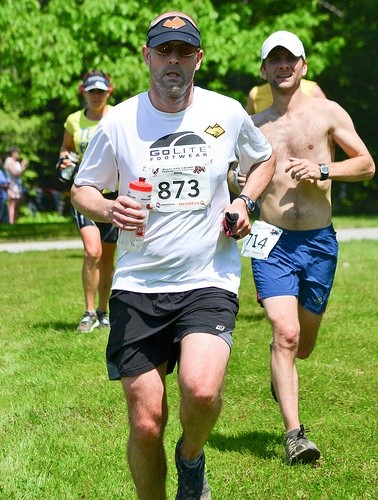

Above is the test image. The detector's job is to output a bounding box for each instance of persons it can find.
[70,11,277,500]
[56,69,119,334]
[227,78,327,197]
[227,31,376,464]
[0,149,28,224]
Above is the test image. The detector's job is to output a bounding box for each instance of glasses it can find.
[148,44,200,56]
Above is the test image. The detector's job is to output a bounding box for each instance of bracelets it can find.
[236,195,256,215]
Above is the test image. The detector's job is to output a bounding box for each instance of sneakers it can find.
[175,436,211,500]
[96,309,110,328]
[284,424,320,464]
[269,337,278,402]
[77,312,100,332]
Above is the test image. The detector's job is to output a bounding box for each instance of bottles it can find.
[117,176,153,253]
[59,152,79,184]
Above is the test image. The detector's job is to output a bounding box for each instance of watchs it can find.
[318,162,330,181]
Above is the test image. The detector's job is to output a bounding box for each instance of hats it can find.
[261,31,305,64]
[146,16,200,47]
[84,76,109,92]
